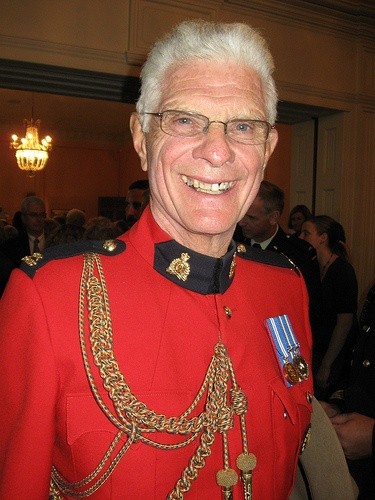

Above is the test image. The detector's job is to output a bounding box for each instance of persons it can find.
[0,207,17,243]
[288,205,312,238]
[298,214,359,401]
[0,197,69,299]
[317,284,375,500]
[12,211,26,233]
[51,180,122,245]
[0,21,314,500]
[125,179,150,231]
[233,181,320,404]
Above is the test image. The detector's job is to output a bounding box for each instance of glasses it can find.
[140,110,275,145]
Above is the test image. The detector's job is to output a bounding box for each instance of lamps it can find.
[10,90,54,179]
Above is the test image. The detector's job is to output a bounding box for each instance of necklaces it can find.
[320,254,334,277]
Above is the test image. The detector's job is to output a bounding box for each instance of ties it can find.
[33,239,40,254]
